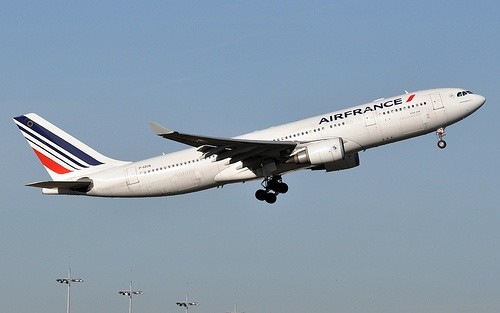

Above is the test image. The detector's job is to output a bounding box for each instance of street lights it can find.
[175,293,200,313]
[118,281,144,313]
[56,267,85,313]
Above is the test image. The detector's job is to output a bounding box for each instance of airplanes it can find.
[11,87,486,204]
